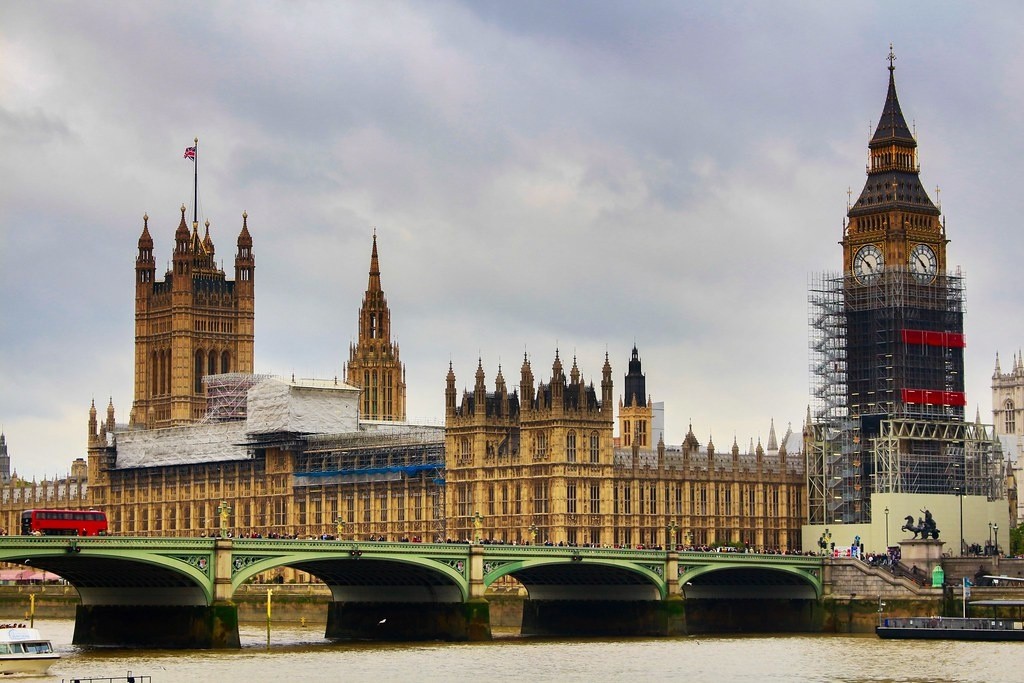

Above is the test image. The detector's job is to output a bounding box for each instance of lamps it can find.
[571,556,583,561]
[350,551,363,556]
[66,546,82,553]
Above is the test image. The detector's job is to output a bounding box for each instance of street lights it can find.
[529,521,539,546]
[822,529,831,556]
[335,516,345,540]
[993,525,999,556]
[667,520,678,551]
[218,500,232,539]
[989,521,992,556]
[471,512,483,544]
[954,487,964,555]
[885,506,889,546]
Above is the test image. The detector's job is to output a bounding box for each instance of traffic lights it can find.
[831,543,834,550]
[971,544,983,554]
[745,539,750,547]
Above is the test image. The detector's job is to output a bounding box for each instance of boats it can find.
[0,626,62,677]
[877,617,1024,642]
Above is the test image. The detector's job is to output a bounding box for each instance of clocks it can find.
[849,243,886,286]
[911,240,940,283]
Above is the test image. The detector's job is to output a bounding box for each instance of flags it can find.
[184,147,195,161]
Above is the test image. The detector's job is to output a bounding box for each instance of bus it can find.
[19,509,109,536]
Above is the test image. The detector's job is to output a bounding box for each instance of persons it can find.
[82,528,87,536]
[921,509,936,539]
[0,623,26,629]
[201,530,1024,586]
[28,528,45,536]
[0,528,5,535]
[73,528,79,535]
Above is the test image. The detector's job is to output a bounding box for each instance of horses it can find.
[901,515,921,539]
